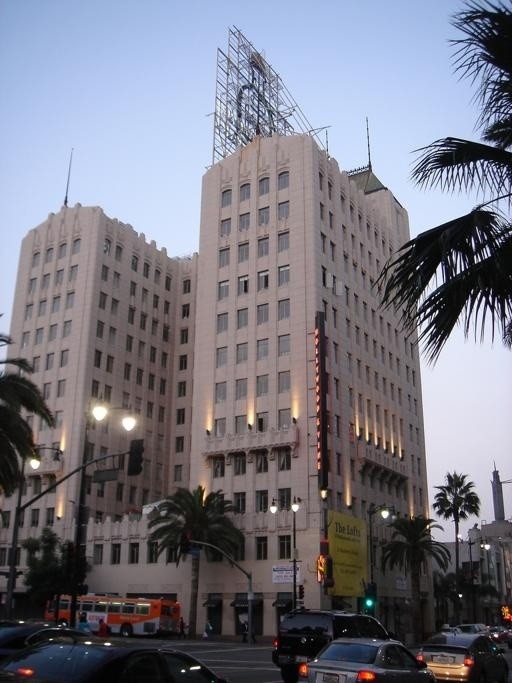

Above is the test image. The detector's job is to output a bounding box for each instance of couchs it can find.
[180,526,194,554]
[365,582,378,616]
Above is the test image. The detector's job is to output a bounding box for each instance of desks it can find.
[468,537,491,623]
[269,496,303,612]
[69,395,142,629]
[7,446,64,586]
[367,504,398,583]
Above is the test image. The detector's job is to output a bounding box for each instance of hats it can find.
[271,604,512,683]
[0,620,230,683]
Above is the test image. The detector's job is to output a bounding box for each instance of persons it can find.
[240,620,248,642]
[97,619,111,637]
[79,612,89,630]
[178,617,185,640]
[205,620,214,639]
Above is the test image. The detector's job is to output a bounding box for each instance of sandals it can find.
[44,593,181,637]
